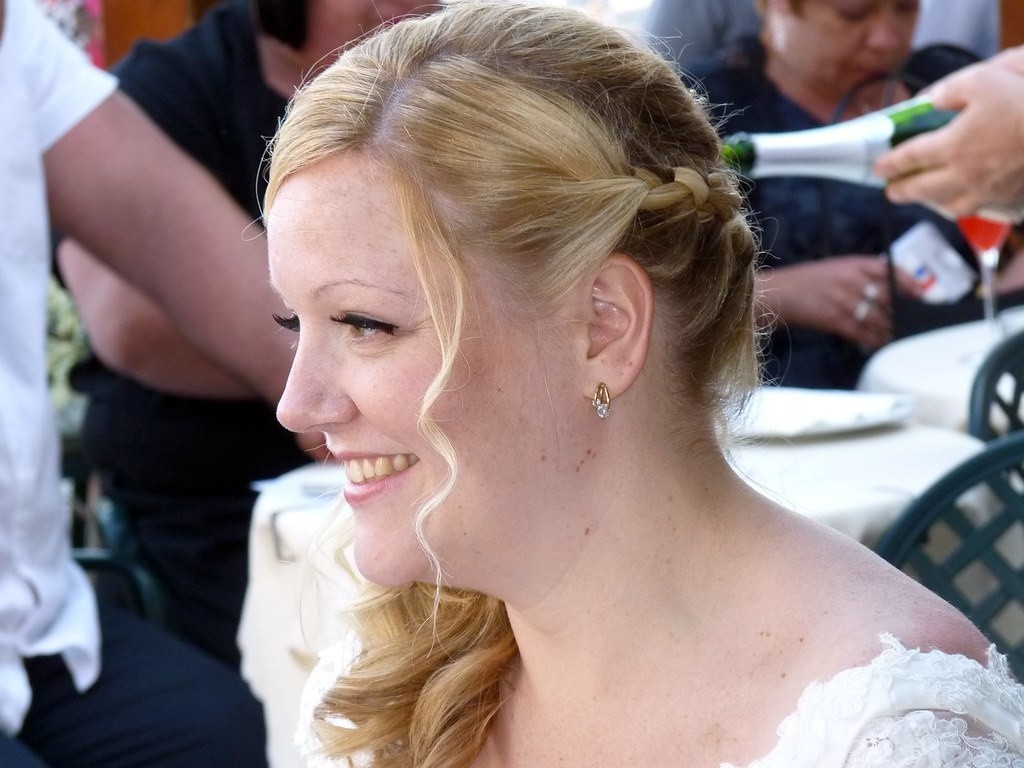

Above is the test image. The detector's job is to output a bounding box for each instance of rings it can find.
[866,285,876,298]
[854,303,867,321]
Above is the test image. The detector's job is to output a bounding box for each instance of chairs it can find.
[878,430,1024,690]
[970,335,1023,440]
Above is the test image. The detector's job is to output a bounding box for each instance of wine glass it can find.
[959,216,1010,322]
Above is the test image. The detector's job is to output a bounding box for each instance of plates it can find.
[723,386,912,439]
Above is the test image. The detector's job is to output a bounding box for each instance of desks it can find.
[237,423,1023,768]
[860,309,1024,433]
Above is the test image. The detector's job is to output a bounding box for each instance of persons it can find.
[260,0,1024,768]
[641,0,1024,393]
[0,0,329,768]
[51,0,448,675]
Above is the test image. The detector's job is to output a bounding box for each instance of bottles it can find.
[721,93,963,190]
